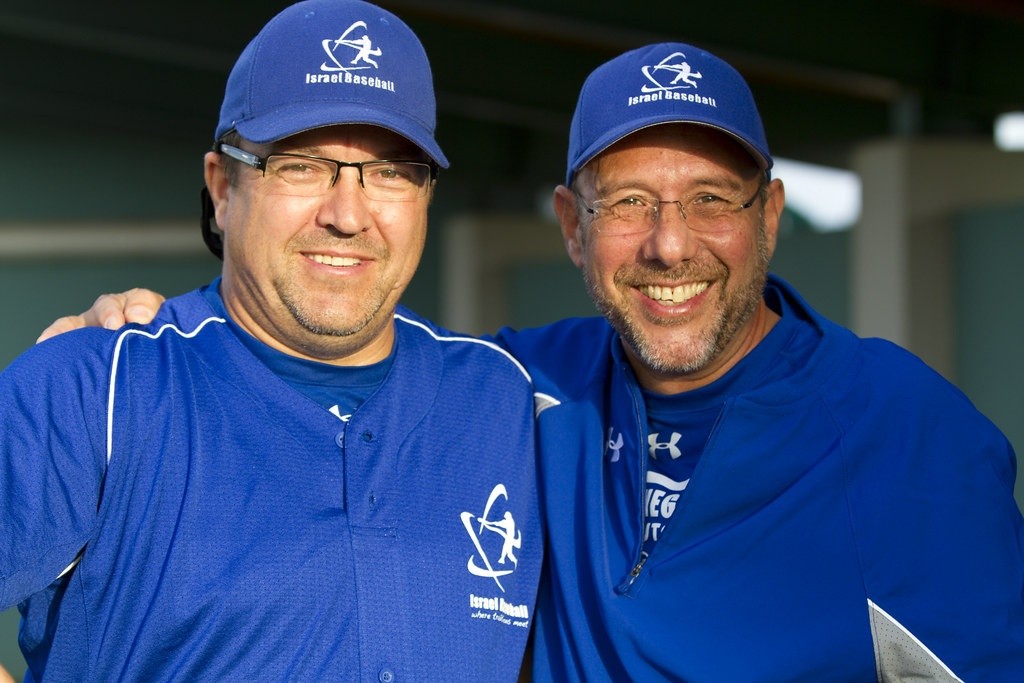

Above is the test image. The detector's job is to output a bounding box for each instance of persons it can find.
[32,35,1024,683]
[0,2,545,683]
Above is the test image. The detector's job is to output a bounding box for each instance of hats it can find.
[215,0,450,169]
[565,42,773,181]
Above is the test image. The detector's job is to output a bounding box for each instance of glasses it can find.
[217,142,437,201]
[573,172,768,236]
